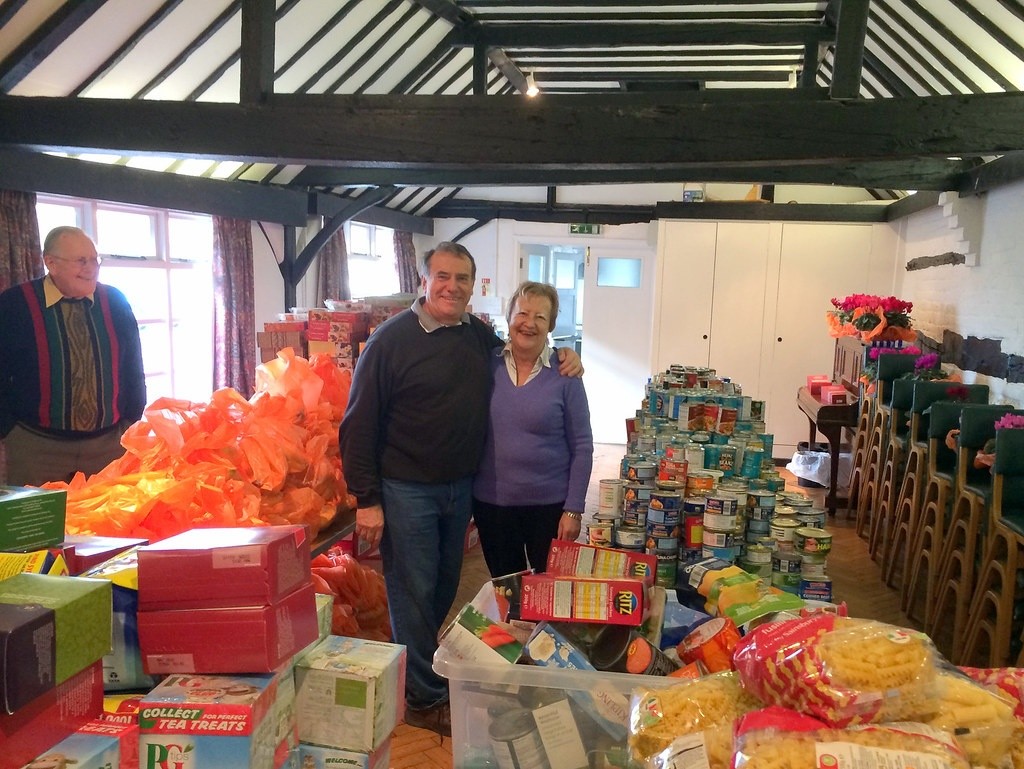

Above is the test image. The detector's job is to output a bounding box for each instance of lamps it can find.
[526,67,539,97]
[789,65,800,88]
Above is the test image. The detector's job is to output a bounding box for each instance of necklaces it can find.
[510,364,539,375]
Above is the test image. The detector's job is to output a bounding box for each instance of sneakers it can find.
[405,701,451,746]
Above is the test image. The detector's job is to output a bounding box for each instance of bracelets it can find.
[566,511,582,521]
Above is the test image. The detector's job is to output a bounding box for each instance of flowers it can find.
[993,412,1024,430]
[946,386,970,402]
[830,293,916,331]
[862,346,922,383]
[899,353,949,381]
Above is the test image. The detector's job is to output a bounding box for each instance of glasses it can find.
[51,255,102,268]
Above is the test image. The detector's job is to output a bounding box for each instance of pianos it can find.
[795,330,903,517]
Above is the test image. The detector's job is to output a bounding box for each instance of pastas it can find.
[625,616,1024,769]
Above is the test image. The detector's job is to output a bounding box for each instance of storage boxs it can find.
[806,374,832,395]
[820,385,844,403]
[431,538,711,769]
[257,295,416,380]
[0,485,407,769]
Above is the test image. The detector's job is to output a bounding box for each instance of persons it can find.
[0,226,147,490]
[469,281,594,576]
[339,239,585,738]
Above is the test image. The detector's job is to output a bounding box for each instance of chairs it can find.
[845,346,1024,669]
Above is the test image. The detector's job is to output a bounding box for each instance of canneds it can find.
[586,364,832,601]
[486,622,711,769]
